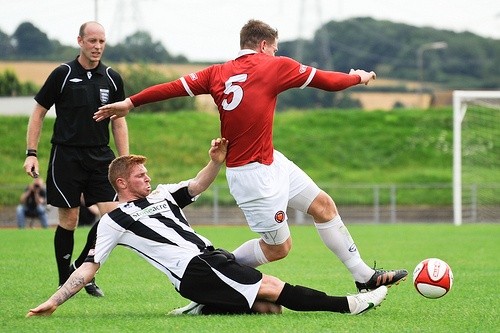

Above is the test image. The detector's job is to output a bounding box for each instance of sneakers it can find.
[69,260,104,296]
[346,286,388,316]
[167,300,204,315]
[355,259,408,293]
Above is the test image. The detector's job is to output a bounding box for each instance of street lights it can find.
[415,41,448,108]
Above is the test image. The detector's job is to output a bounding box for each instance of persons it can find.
[22,21,130,297]
[16,178,50,230]
[92,19,409,293]
[25,138,389,319]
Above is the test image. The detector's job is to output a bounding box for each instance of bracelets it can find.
[27,153,37,157]
[26,149,37,155]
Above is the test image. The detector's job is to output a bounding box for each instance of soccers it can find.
[412,258,454,299]
